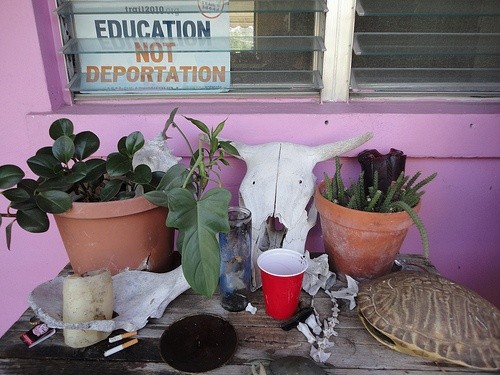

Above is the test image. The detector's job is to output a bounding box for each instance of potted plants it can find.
[314,155,441,288]
[0,118,244,306]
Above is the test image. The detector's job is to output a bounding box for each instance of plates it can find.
[159,313,239,374]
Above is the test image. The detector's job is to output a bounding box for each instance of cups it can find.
[257,247,308,319]
[217,207,254,312]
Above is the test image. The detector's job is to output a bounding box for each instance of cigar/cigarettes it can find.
[109,331,138,344]
[104,337,138,357]
